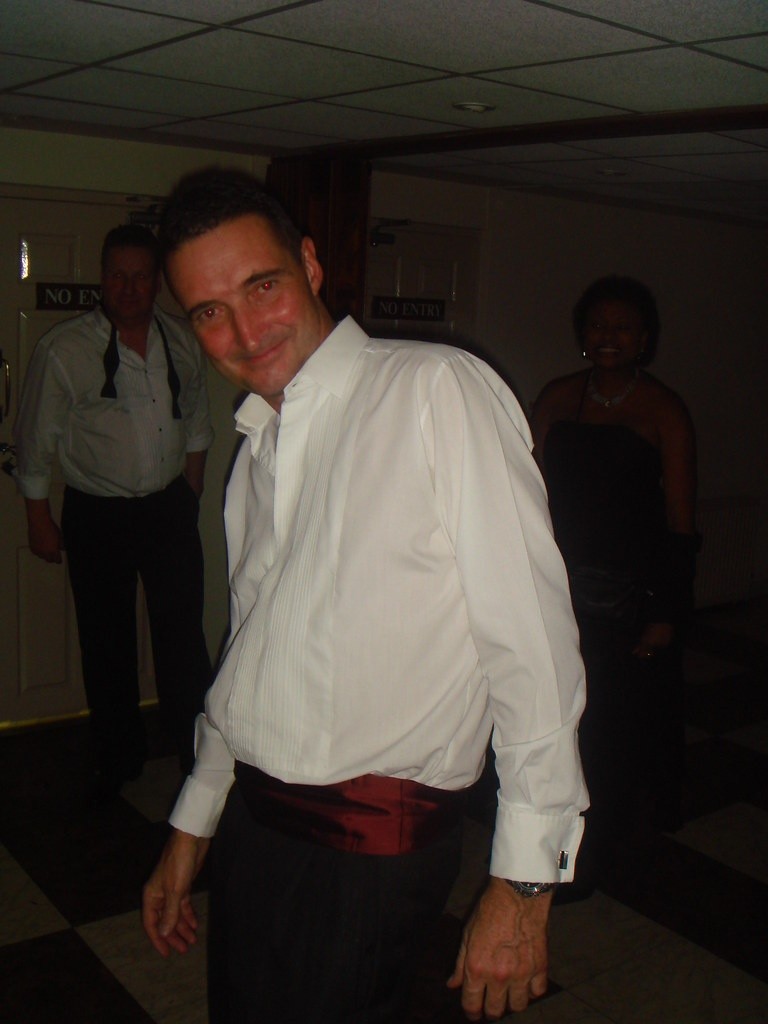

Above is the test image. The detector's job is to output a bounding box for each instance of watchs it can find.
[506,880,566,897]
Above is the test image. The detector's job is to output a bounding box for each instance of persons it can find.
[142,167,591,1024]
[14,224,216,806]
[529,276,705,833]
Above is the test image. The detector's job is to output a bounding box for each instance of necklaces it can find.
[585,363,639,408]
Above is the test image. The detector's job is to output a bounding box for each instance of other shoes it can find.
[178,742,195,778]
[106,726,141,782]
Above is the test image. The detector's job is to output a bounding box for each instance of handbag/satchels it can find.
[573,569,649,625]
